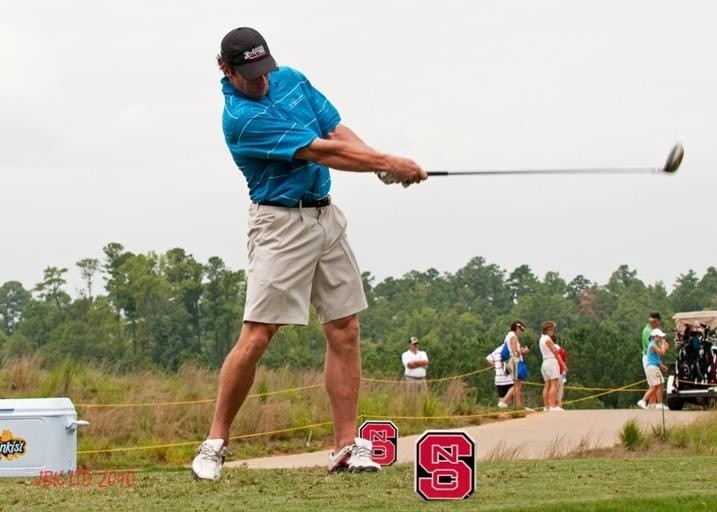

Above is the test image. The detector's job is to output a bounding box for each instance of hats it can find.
[650,329,666,337]
[514,322,526,332]
[408,336,420,344]
[220,27,279,80]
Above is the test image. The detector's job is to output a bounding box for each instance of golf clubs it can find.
[377,143,684,180]
[673,323,717,347]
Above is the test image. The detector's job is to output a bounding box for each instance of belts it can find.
[406,375,425,380]
[254,194,332,208]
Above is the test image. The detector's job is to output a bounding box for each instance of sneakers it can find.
[637,400,647,410]
[647,402,669,410]
[327,437,382,474]
[544,406,565,412]
[497,402,508,408]
[191,437,230,481]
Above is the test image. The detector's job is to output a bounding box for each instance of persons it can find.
[504,318,531,408]
[538,320,564,411]
[551,335,568,406]
[484,337,513,408]
[641,311,669,410]
[190,24,429,483]
[400,335,429,392]
[636,328,670,410]
